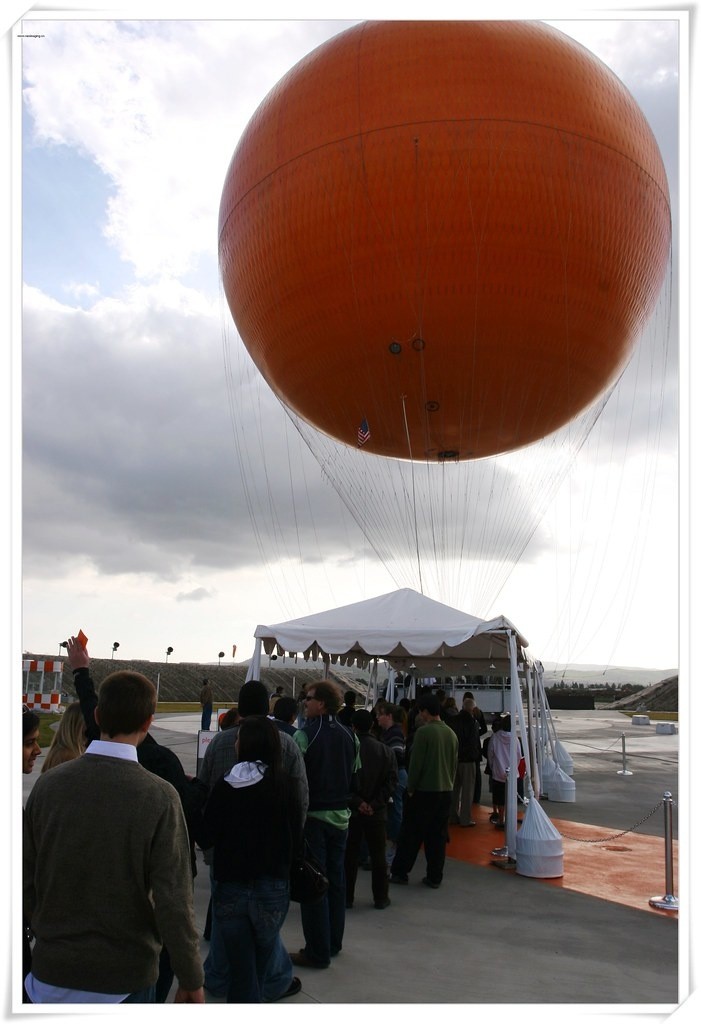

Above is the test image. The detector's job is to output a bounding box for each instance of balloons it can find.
[219,19,673,463]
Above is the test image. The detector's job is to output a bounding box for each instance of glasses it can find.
[306,696,315,701]
[23,704,29,714]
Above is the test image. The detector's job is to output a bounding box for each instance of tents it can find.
[244,588,576,879]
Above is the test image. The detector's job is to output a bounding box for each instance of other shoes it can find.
[490,813,500,823]
[289,952,328,969]
[387,873,409,885]
[275,976,302,1001]
[299,948,331,964]
[422,877,440,888]
[374,898,391,909]
[462,821,476,826]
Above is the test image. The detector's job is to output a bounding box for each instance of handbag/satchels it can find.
[289,839,329,908]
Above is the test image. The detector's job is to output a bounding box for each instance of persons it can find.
[199,679,214,732]
[22,630,526,1004]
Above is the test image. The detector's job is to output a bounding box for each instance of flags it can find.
[355,418,372,450]
